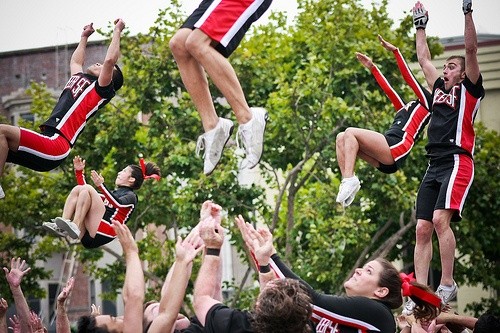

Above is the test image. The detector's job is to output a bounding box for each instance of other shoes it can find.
[0,185,5,198]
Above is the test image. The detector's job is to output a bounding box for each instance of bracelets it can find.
[259,265,271,274]
[206,248,220,256]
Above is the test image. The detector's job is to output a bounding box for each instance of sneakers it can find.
[52,217,81,239]
[336,176,360,203]
[402,300,416,316]
[196,116,234,176]
[42,221,68,236]
[435,280,457,310]
[343,186,361,207]
[235,106,267,169]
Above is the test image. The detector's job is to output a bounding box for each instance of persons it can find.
[42,154,160,252]
[0,256,75,333]
[168,0,273,177]
[0,14,126,201]
[75,201,223,332]
[397,303,500,333]
[412,0,485,307]
[191,199,311,333]
[333,33,435,208]
[233,209,449,333]
